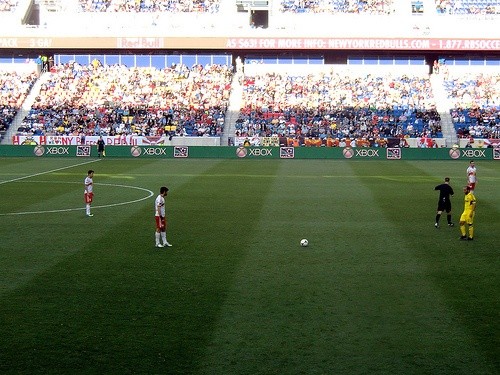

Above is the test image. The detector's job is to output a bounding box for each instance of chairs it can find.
[244,107,296,125]
[451,109,500,138]
[377,105,442,137]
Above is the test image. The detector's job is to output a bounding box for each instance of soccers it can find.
[298,238,309,246]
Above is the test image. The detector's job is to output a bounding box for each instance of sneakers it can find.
[447,222,455,227]
[464,237,474,241]
[434,222,440,230]
[457,235,468,241]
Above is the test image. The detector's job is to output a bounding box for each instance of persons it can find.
[433,178,455,228]
[155,187,172,248]
[84,170,95,217]
[458,185,476,240]
[0,0,500,199]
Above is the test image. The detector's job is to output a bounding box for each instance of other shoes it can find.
[154,243,164,248]
[163,242,173,247]
[97,157,102,159]
[86,213,94,217]
